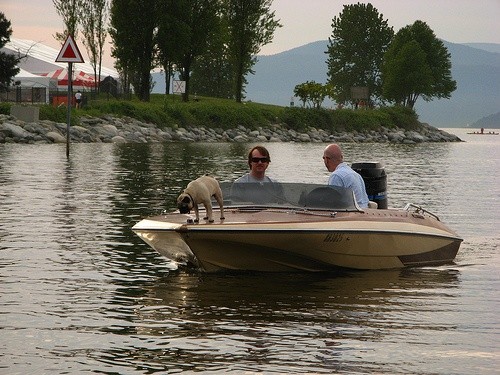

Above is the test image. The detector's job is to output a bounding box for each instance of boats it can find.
[128,161,465,277]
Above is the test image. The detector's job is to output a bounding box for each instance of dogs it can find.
[175,173,226,223]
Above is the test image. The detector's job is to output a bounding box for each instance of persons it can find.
[231,147,284,203]
[323,144,368,210]
[74,90,81,108]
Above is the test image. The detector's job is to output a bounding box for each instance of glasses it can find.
[252,158,268,163]
